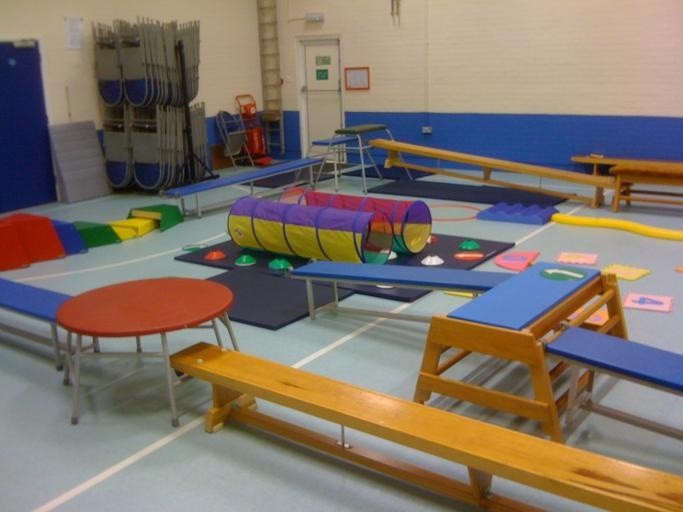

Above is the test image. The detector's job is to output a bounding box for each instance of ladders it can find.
[257,0,286,153]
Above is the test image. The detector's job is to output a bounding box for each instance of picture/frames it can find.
[344,66,370,91]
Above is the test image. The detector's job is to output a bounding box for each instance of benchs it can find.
[0,279,75,372]
[412,252,627,443]
[291,257,520,322]
[159,153,325,220]
[166,340,680,512]
[545,322,682,442]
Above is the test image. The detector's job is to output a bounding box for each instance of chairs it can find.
[90,14,209,193]
[214,109,254,169]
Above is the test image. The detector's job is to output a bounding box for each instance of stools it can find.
[305,123,414,193]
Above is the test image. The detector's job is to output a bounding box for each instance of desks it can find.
[51,274,239,427]
